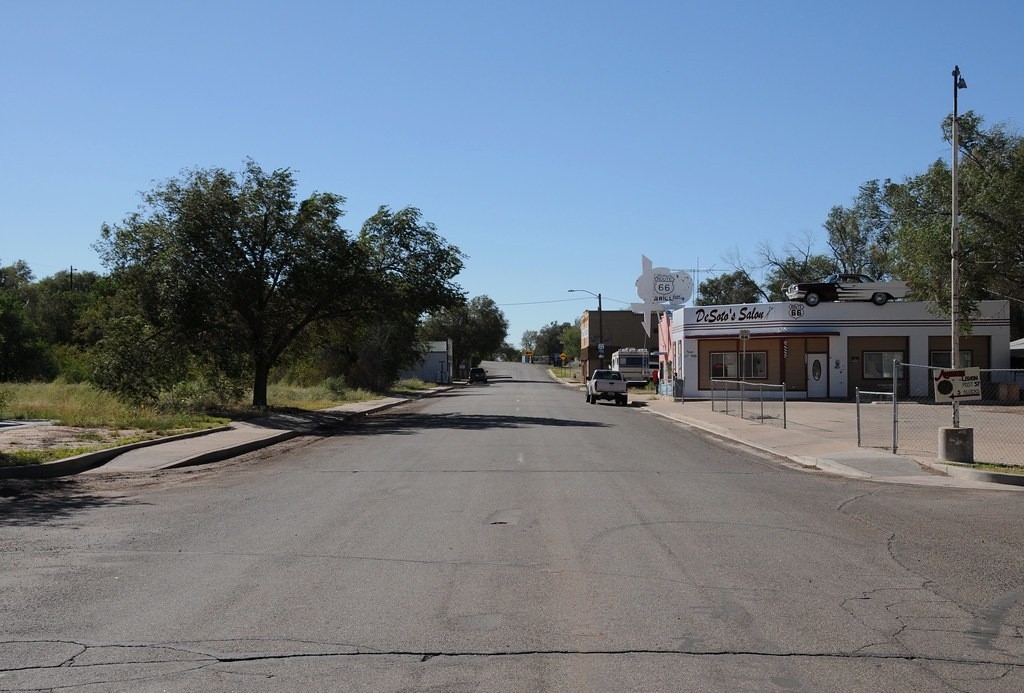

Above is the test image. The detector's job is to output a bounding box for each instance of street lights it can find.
[568,290,604,369]
[951,64,967,429]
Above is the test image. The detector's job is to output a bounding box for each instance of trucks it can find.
[608,348,650,388]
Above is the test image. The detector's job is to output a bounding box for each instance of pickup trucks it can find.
[585,369,628,407]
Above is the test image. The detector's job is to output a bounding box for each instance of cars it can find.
[649,361,659,380]
[469,368,488,384]
[786,274,914,306]
